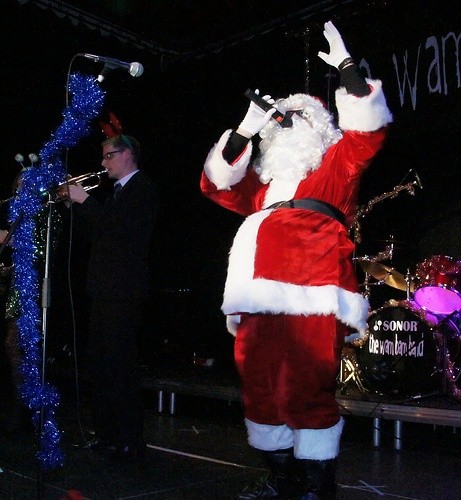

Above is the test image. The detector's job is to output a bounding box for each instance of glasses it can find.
[101,149,134,160]
[285,110,303,117]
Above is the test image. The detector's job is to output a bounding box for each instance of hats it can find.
[273,94,333,122]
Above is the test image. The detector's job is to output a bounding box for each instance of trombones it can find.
[0,167,110,218]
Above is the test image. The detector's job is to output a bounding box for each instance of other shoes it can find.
[106,438,128,455]
[71,433,109,449]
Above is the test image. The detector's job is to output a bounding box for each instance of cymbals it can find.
[358,258,414,293]
[376,239,405,244]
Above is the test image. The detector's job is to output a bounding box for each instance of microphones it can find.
[244,88,293,128]
[78,53,144,77]
[415,171,424,188]
[371,367,397,374]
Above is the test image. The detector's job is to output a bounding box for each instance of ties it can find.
[113,183,122,199]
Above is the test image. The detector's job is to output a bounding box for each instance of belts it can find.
[263,199,348,228]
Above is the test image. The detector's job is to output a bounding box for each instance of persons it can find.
[198,22,395,500]
[62,135,169,476]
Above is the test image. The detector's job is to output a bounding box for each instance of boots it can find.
[237,416,299,500]
[285,416,345,500]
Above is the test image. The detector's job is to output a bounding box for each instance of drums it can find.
[414,254,461,315]
[351,299,461,400]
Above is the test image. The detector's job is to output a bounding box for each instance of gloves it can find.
[317,21,354,70]
[238,90,278,136]
